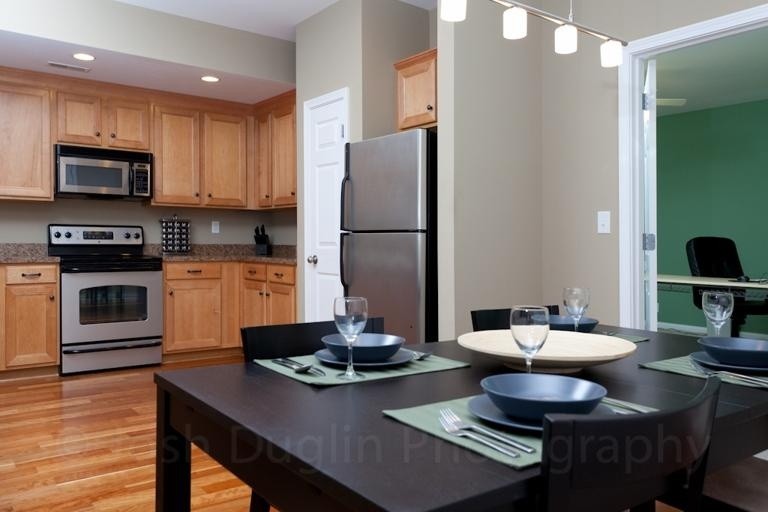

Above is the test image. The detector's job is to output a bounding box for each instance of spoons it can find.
[272,358,314,373]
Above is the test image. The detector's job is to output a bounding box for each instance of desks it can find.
[655,272,768,299]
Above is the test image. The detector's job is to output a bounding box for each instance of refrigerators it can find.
[339,127,438,343]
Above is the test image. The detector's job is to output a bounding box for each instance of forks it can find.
[439,408,536,454]
[414,352,431,360]
[438,415,521,458]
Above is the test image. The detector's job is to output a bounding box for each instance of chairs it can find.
[465,304,562,331]
[685,233,768,337]
[235,315,389,512]
[655,451,768,511]
[533,375,722,512]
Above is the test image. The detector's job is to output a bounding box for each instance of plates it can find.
[457,329,638,374]
[467,394,619,434]
[689,351,768,372]
[315,346,414,367]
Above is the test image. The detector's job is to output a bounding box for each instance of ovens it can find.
[54,143,153,201]
[62,271,163,346]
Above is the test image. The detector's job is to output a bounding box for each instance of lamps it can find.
[435,0,630,71]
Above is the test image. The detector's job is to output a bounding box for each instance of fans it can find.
[655,98,687,108]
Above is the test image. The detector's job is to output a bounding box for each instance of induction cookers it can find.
[61,255,161,270]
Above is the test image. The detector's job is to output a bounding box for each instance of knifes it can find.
[281,357,326,376]
[253,224,274,256]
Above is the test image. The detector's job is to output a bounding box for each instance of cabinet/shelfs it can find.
[236,255,297,347]
[0,257,60,380]
[0,67,56,204]
[146,87,251,211]
[49,73,155,154]
[161,258,239,356]
[251,84,298,210]
[392,46,437,134]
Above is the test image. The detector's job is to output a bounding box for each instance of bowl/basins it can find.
[321,333,407,362]
[696,334,768,365]
[481,373,609,422]
[531,313,598,332]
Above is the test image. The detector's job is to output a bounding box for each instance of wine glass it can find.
[701,290,735,336]
[333,296,368,380]
[564,286,589,329]
[510,305,551,373]
